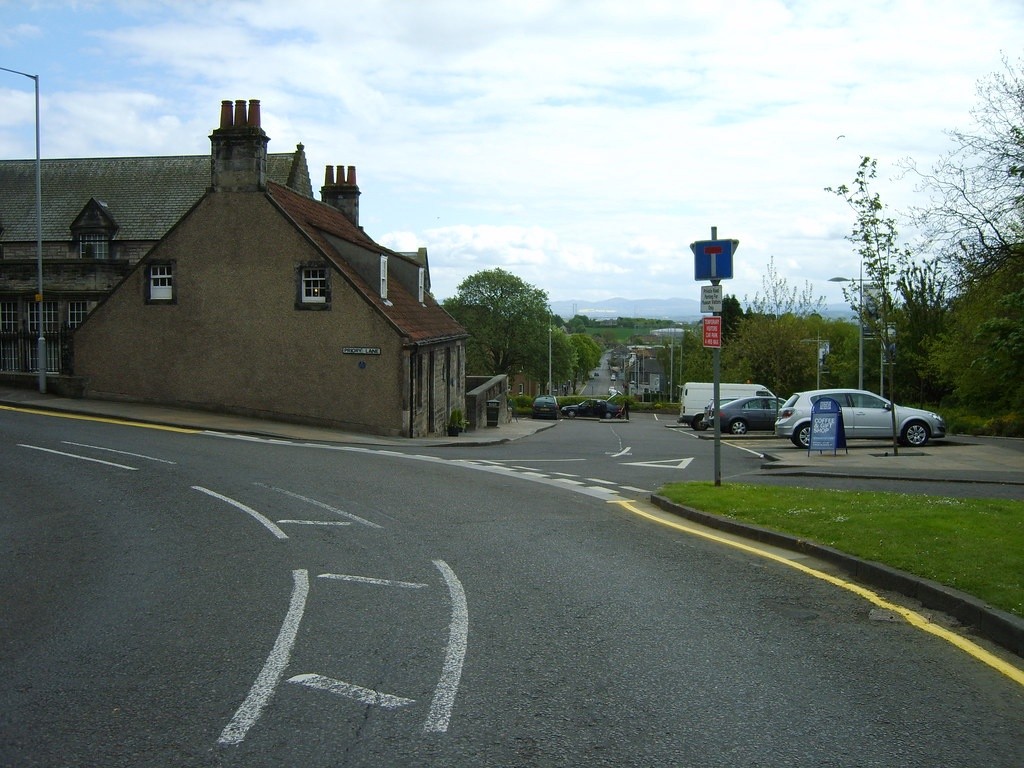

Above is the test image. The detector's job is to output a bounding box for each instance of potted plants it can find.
[447,409,467,436]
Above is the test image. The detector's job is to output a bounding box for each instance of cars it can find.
[560,399,624,419]
[595,372,599,377]
[589,375,595,380]
[532,395,558,420]
[774,388,948,450]
[708,397,788,435]
[610,374,616,381]
[700,398,736,427]
[608,386,618,396]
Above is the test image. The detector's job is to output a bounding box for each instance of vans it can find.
[676,382,788,431]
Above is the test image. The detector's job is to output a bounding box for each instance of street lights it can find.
[799,329,823,389]
[829,277,885,399]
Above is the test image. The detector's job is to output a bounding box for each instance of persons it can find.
[623,398,630,420]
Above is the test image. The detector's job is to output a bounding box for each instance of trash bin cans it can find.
[486,400,500,426]
